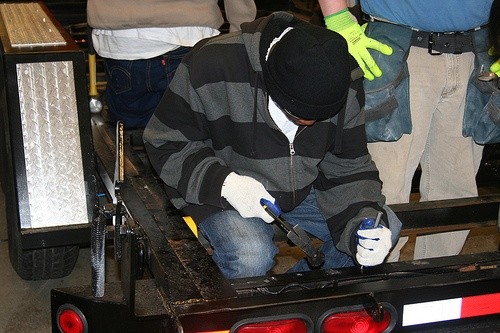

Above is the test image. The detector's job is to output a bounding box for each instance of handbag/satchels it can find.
[462,28,500,145]
[362,21,412,143]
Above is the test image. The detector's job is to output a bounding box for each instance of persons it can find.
[143,11,402,290]
[317,0,500,263]
[88,0,256,137]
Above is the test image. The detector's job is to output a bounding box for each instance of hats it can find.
[266,26,350,120]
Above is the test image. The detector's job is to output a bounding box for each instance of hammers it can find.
[260,200,327,270]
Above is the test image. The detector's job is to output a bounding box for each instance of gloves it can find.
[488,42,500,78]
[221,171,281,223]
[354,217,392,266]
[323,8,393,82]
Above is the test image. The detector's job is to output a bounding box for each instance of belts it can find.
[164,45,194,55]
[413,28,476,55]
